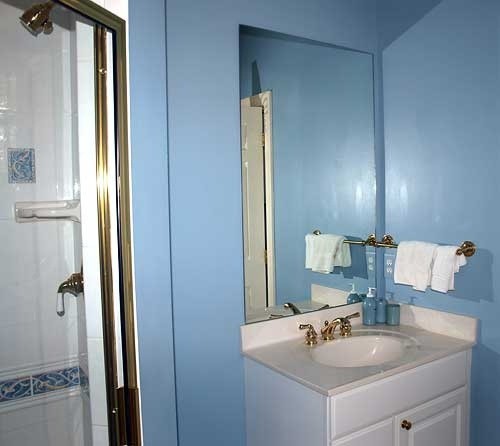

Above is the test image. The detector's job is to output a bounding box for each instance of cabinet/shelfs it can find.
[238,295,480,446]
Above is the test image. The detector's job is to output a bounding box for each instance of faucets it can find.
[321,318,352,340]
[284,303,300,315]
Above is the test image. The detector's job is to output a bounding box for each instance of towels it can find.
[393,240,465,292]
[303,230,352,271]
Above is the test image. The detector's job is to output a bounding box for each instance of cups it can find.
[387,304,400,326]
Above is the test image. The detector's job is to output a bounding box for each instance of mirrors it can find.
[238,23,376,325]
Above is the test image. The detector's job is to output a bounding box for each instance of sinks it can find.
[311,329,419,368]
